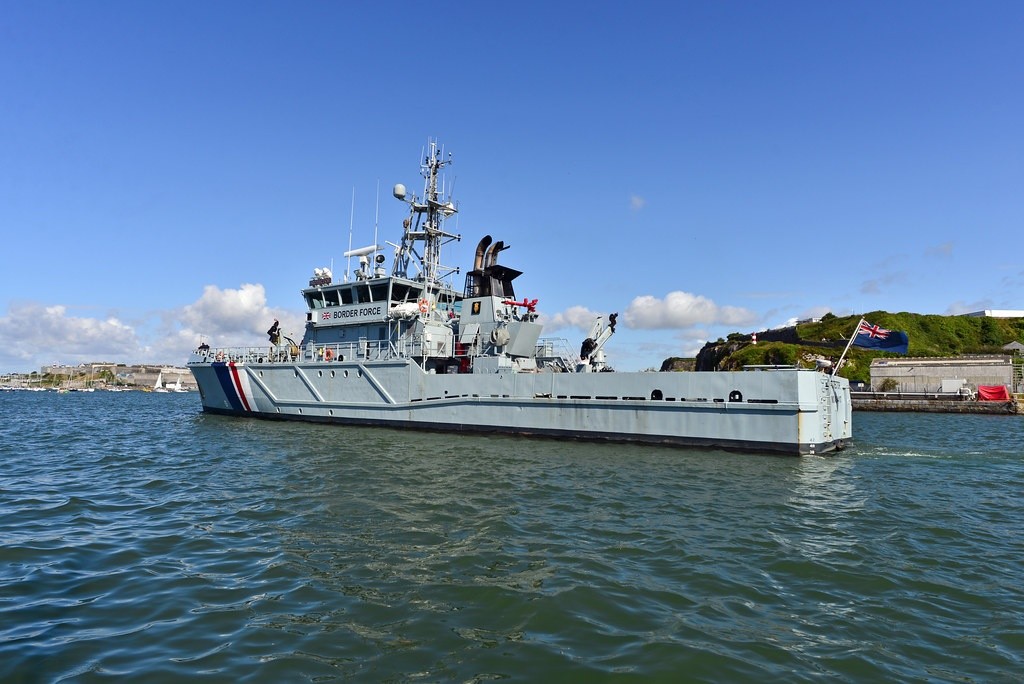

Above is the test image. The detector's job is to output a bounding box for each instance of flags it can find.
[849,319,908,355]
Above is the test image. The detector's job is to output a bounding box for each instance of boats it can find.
[185,137,852,457]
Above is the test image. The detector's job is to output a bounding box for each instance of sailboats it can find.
[0,368,190,393]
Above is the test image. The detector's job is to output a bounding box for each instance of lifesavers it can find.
[325,349,334,361]
[419,299,430,313]
[217,351,224,360]
[450,311,454,318]
